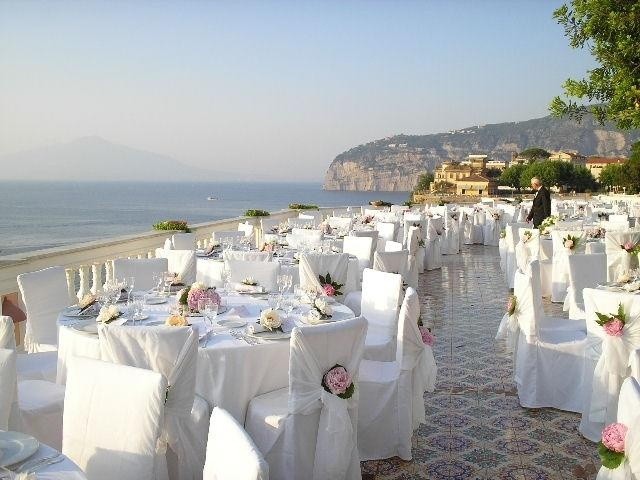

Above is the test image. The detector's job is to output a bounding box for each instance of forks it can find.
[231,330,259,346]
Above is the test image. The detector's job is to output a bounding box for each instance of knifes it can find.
[202,333,210,349]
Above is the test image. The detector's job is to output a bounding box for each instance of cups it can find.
[327,190,476,242]
[508,190,640,243]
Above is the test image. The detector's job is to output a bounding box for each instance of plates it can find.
[73,319,117,334]
[142,297,167,306]
[217,318,249,328]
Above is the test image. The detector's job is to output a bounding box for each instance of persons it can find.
[525,176,551,228]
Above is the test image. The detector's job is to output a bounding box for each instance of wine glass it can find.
[206,232,344,263]
[100,273,333,332]
